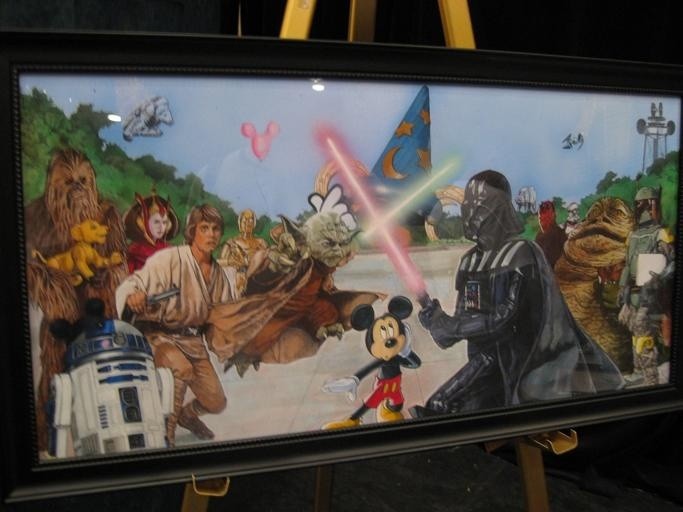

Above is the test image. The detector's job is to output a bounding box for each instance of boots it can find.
[175,397,217,441]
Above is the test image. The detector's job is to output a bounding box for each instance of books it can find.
[635,253,667,288]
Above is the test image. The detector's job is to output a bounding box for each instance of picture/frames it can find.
[0,28,683,504]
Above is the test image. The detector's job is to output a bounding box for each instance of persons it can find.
[612,186,675,390]
[113,203,241,449]
[407,170,544,419]
[121,182,179,275]
[534,201,568,270]
[222,207,268,294]
[557,202,581,240]
